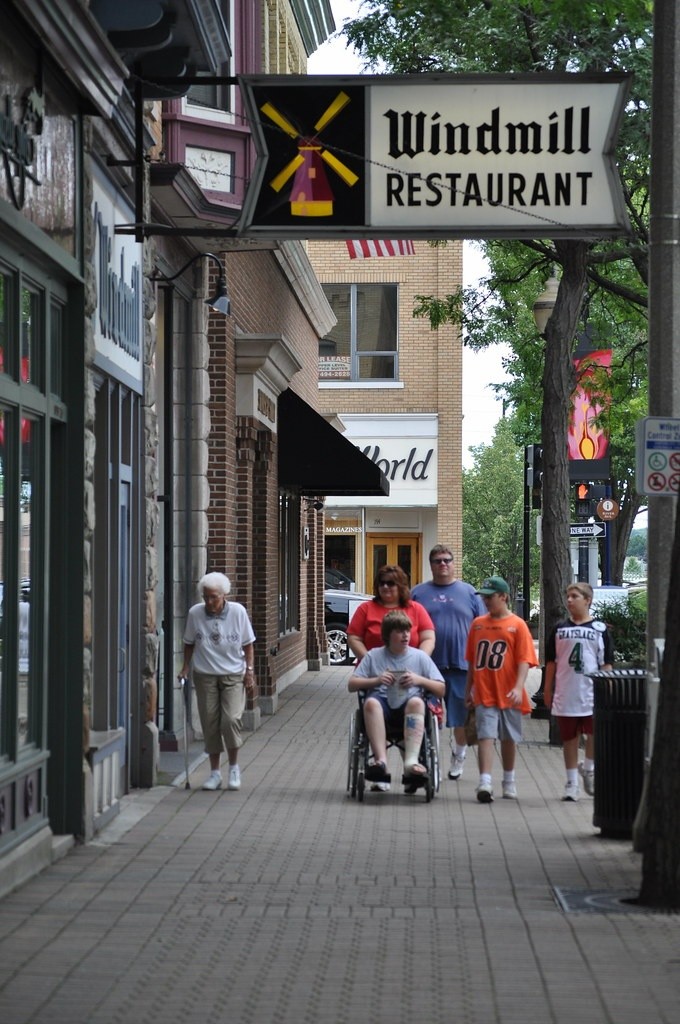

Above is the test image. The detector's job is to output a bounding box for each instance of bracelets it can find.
[246,666,254,671]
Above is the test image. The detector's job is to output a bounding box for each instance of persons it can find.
[348,565,436,792]
[544,581,614,802]
[348,610,446,786]
[175,571,256,790]
[409,543,488,781]
[465,576,539,803]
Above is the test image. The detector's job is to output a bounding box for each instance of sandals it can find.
[370,760,386,776]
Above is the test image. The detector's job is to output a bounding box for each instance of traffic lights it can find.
[575,483,592,516]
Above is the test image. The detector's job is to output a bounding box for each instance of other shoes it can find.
[578,763,595,796]
[371,782,390,792]
[502,780,516,798]
[476,784,494,803]
[404,783,417,794]
[448,754,465,779]
[562,782,581,801]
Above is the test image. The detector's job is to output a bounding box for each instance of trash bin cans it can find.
[584,669,648,839]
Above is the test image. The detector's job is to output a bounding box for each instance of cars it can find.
[324,580,376,666]
[325,568,354,591]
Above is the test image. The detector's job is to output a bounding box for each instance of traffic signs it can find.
[570,523,606,539]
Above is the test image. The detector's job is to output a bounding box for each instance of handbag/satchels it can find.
[465,709,478,746]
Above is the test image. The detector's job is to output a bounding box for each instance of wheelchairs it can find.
[347,685,442,804]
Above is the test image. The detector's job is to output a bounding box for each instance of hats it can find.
[474,577,510,595]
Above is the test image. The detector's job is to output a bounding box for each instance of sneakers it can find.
[228,769,241,790]
[202,775,223,790]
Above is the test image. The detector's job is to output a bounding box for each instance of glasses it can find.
[380,580,395,587]
[433,558,453,564]
